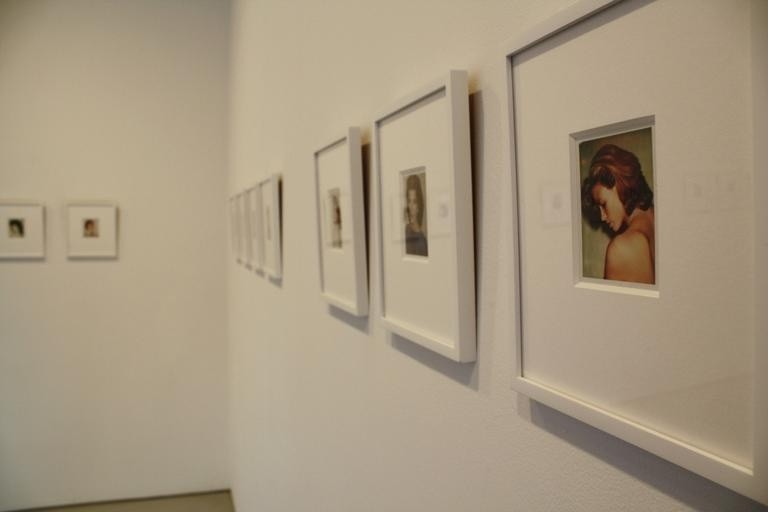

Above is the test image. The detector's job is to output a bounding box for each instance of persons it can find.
[401,174,427,256]
[579,144,654,287]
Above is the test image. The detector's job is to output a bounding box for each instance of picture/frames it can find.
[0,199,47,260]
[368,69,478,362]
[498,0,768,507]
[226,174,284,286]
[65,200,118,260]
[309,125,369,317]
